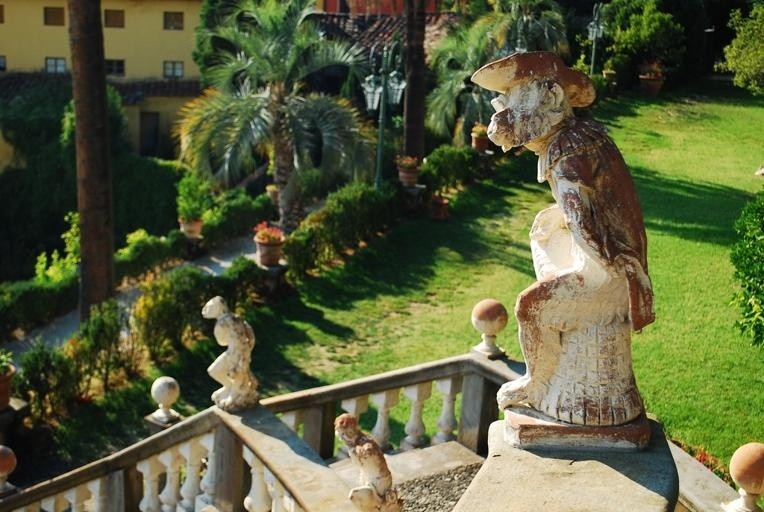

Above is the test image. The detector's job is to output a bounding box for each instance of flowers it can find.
[474,122,488,134]
[398,156,419,167]
[254,221,285,240]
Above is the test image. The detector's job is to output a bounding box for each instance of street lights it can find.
[363,39,408,189]
[586,2,604,76]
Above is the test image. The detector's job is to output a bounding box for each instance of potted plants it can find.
[628,0,685,99]
[419,143,472,221]
[601,52,628,80]
[0,351,16,408]
[174,170,217,238]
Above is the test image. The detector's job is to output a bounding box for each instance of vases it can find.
[252,237,287,266]
[398,167,419,187]
[470,133,491,153]
